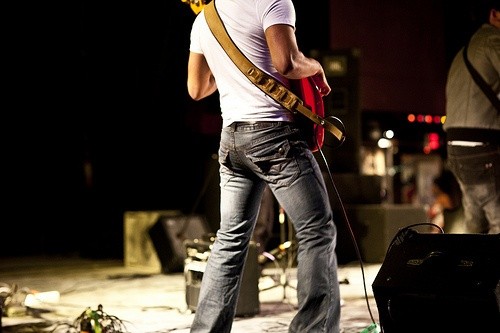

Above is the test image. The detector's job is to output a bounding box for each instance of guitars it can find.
[181,0,324,152]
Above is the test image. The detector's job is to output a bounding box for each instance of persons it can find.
[187,0,341,333]
[431,0,500,234]
